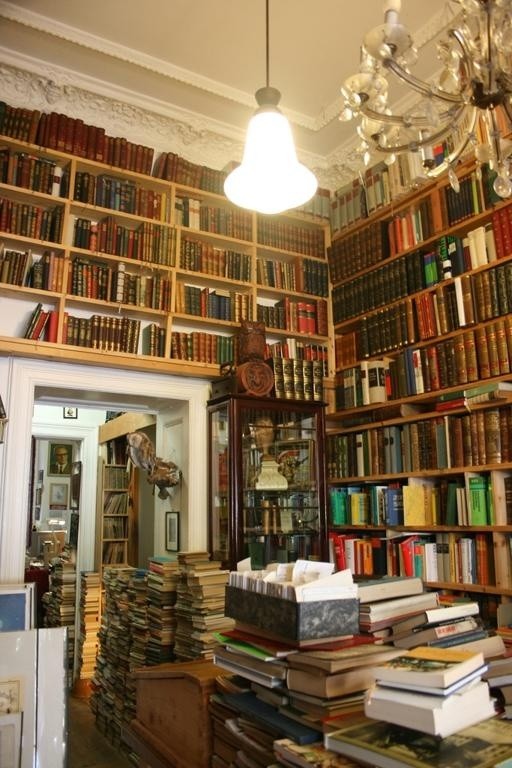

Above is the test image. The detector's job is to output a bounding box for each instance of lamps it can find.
[340,0,512,198]
[224,1,319,215]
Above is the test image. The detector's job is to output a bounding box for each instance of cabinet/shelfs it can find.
[93,455,136,629]
[205,392,326,572]
[2,130,332,397]
[326,152,512,654]
